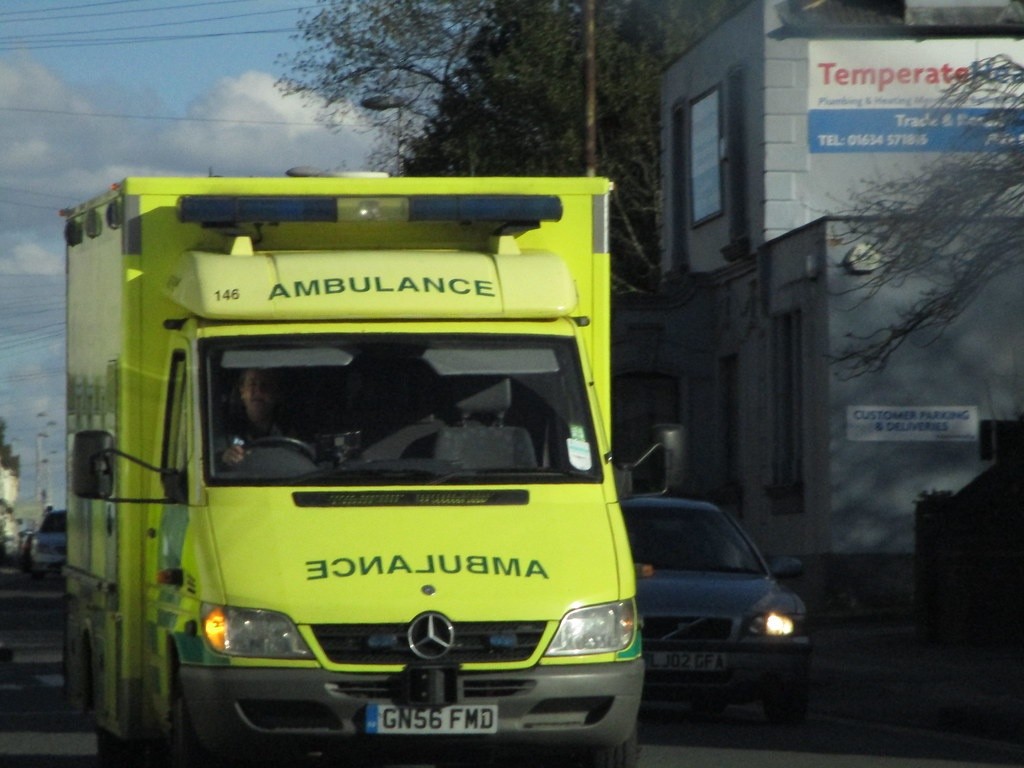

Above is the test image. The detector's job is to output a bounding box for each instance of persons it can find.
[217,364,325,470]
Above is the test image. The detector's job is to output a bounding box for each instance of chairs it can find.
[434,368,538,473]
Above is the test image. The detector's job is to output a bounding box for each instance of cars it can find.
[31,508,64,580]
[614,494,807,726]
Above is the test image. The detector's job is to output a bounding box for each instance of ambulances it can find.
[58,173,641,768]
